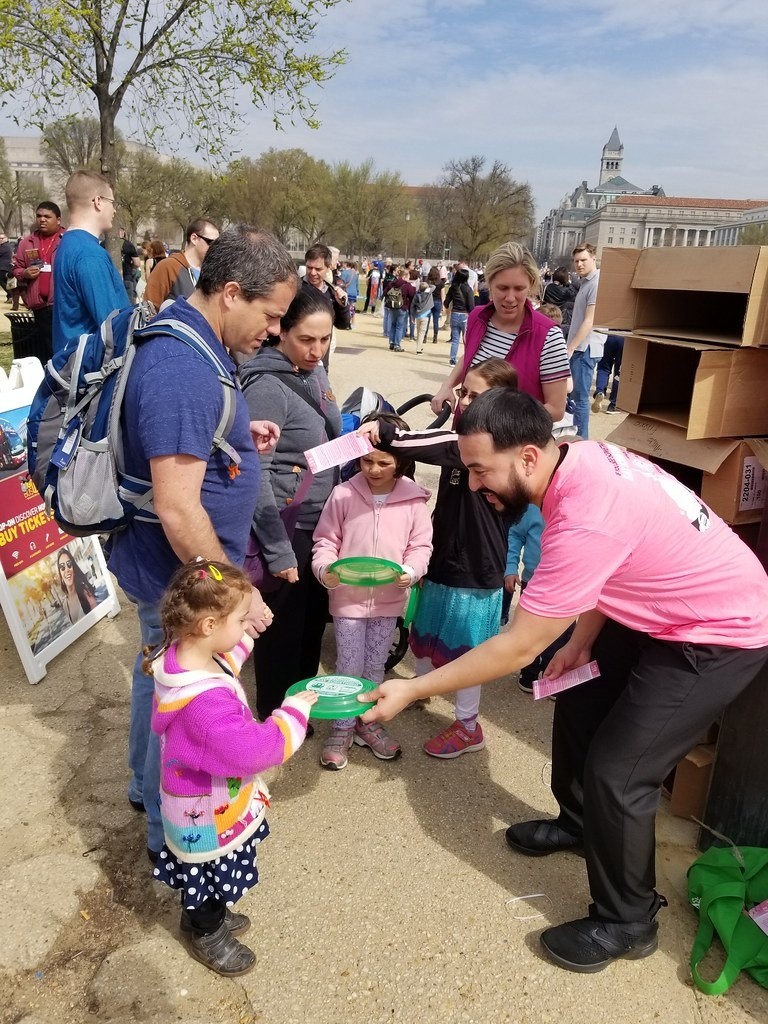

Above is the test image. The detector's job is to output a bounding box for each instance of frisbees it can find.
[286,674,378,719]
[330,556,403,585]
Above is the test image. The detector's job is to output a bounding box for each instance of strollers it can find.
[335,384,453,483]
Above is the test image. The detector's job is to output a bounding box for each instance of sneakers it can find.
[421,719,486,759]
[319,716,403,772]
[591,391,623,415]
[540,889,670,974]
[505,818,587,860]
[518,656,556,701]
[179,906,257,977]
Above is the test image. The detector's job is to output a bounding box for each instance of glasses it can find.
[455,387,478,404]
[91,196,115,207]
[58,559,73,571]
[196,234,215,245]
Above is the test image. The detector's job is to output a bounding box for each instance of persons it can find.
[105,227,301,862]
[143,219,220,313]
[531,244,624,438]
[99,227,167,304]
[412,242,570,623]
[303,245,359,375]
[358,388,768,974]
[142,558,318,977]
[362,254,490,365]
[240,286,342,739]
[53,169,128,360]
[0,201,65,359]
[58,548,98,625]
[356,358,528,757]
[311,413,433,769]
[505,501,577,700]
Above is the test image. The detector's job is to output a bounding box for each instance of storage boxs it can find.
[590,245,768,824]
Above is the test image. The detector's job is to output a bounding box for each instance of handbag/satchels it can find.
[243,501,301,590]
[5,276,17,290]
[686,845,768,996]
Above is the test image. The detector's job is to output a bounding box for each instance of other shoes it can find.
[4,298,26,311]
[128,796,146,813]
[256,705,315,739]
[388,334,456,369]
[146,847,160,863]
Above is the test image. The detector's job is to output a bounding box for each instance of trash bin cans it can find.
[4,312,37,359]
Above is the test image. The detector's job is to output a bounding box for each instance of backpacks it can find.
[26,277,243,538]
[383,284,408,309]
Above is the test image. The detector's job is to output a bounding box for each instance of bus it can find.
[1,417,27,472]
